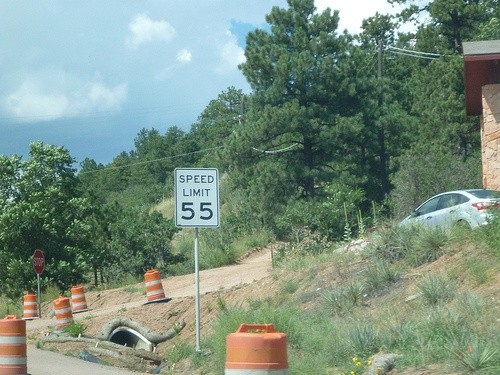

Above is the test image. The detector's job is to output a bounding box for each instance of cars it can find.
[398,188,500,233]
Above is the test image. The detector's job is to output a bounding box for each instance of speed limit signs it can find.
[173,167,222,229]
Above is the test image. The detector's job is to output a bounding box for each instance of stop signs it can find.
[32,250,44,274]
